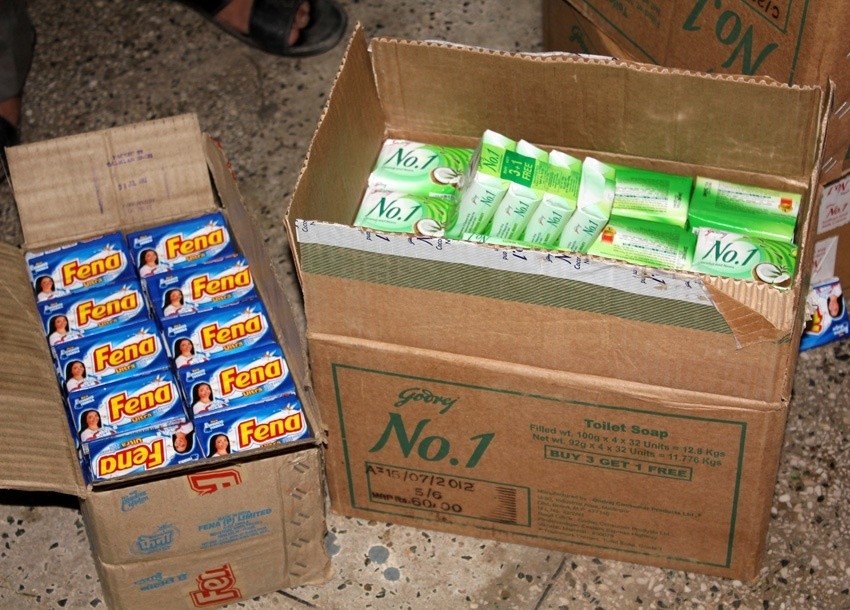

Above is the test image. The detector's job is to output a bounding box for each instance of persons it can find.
[36,276,64,301]
[816,282,843,317]
[160,422,195,453]
[79,410,116,442]
[48,315,83,346]
[0,0,348,185]
[66,361,100,392]
[139,249,171,277]
[175,338,208,368]
[207,434,230,458]
[163,289,196,317]
[190,382,226,415]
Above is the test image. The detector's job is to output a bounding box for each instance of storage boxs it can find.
[0,110,335,610]
[281,17,837,585]
[540,0,850,304]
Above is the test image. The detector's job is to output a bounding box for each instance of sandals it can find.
[177,0,349,59]
[0,97,24,183]
